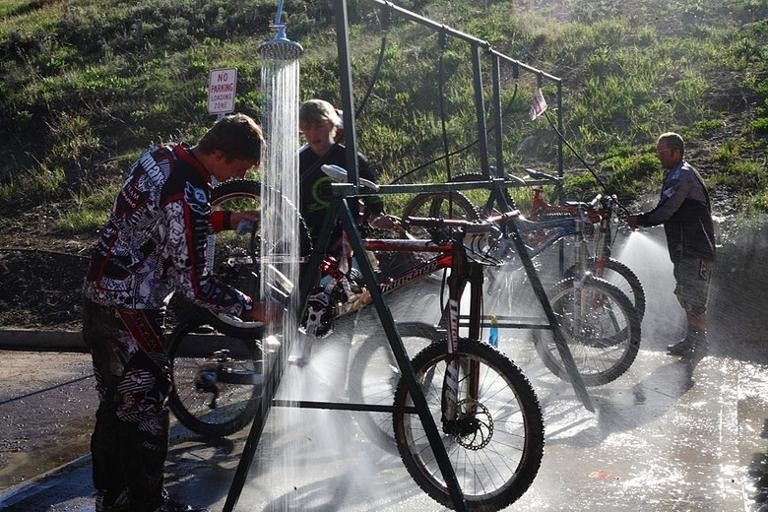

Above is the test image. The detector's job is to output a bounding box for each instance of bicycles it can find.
[157,208,546,512]
[175,164,647,392]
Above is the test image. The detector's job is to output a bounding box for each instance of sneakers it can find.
[666,331,693,354]
[679,332,711,364]
[152,498,188,511]
[91,487,169,512]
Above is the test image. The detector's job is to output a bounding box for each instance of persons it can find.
[331,105,349,144]
[625,131,717,379]
[290,97,381,257]
[81,111,268,510]
